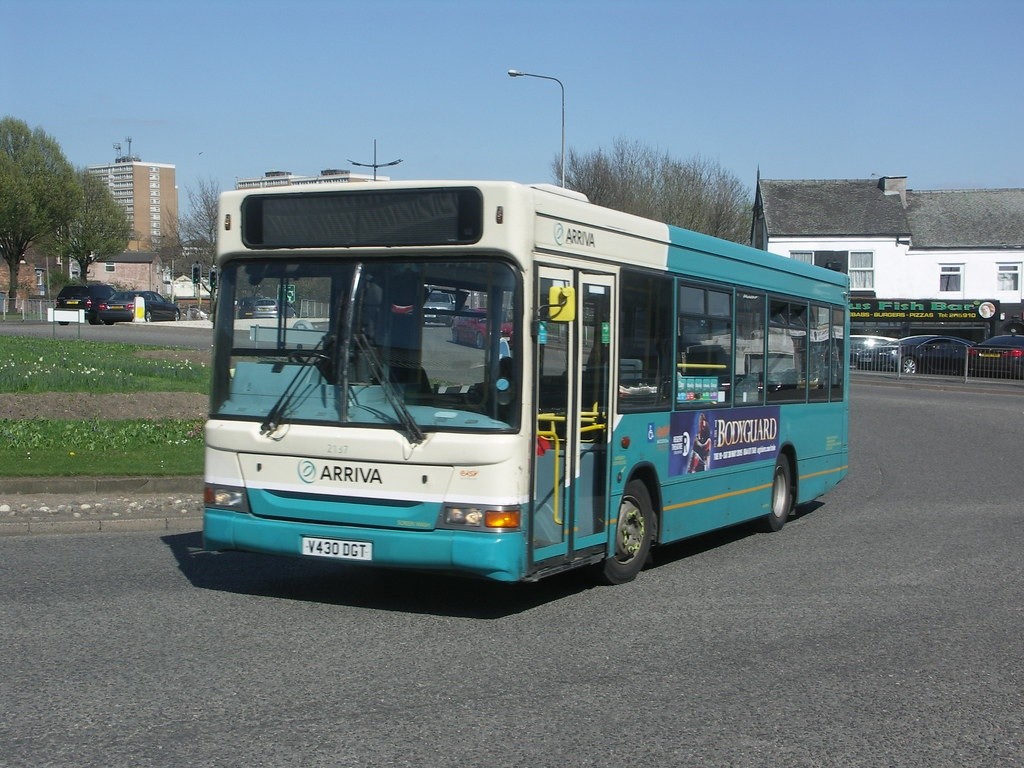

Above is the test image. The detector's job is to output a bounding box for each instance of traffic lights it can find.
[192,264,201,283]
[209,268,218,287]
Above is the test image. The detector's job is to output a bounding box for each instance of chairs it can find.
[619,359,642,378]
[685,345,730,376]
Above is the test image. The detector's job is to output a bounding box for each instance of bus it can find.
[202,180,850,587]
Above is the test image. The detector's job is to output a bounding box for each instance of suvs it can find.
[422,290,456,327]
[54,283,117,325]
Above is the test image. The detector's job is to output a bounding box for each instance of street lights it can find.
[508,69,566,188]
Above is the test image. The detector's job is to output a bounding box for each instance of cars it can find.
[451,307,513,349]
[851,335,1024,380]
[97,290,180,326]
[252,298,296,319]
